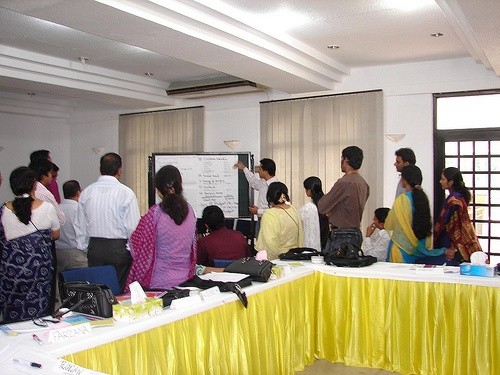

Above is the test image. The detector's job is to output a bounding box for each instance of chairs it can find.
[58,265,122,306]
[210,258,235,269]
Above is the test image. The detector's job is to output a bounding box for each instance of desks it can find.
[0,256,500,375]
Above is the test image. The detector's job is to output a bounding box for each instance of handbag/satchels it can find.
[326,255,377,268]
[279,248,319,260]
[53,281,114,319]
[223,257,273,283]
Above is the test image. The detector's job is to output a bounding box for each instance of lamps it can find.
[223,139,242,149]
[384,133,406,144]
[91,146,105,155]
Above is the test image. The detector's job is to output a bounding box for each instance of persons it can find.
[0,149,330,326]
[319,146,372,258]
[361,147,484,264]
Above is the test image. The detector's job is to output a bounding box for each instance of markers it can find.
[32,333,44,346]
[12,358,42,368]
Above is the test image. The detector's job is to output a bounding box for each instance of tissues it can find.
[111,280,164,324]
[459,251,498,278]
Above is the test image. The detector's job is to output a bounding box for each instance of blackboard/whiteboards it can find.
[148,151,256,222]
